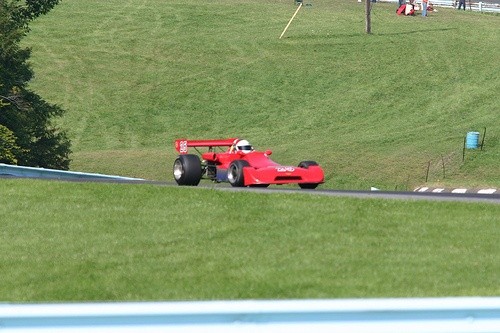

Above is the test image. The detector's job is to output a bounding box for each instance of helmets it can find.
[236,139,252,154]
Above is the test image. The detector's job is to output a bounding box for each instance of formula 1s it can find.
[174,137,325,191]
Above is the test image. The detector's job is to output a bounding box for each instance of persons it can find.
[235,139,253,154]
[458,0,466,11]
